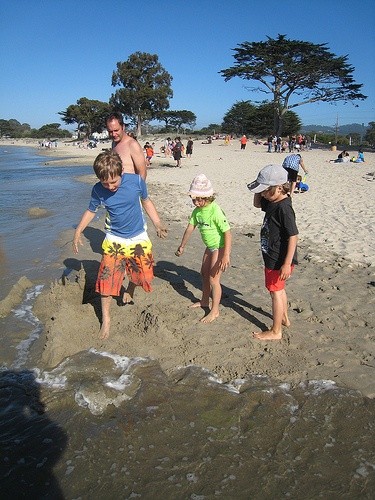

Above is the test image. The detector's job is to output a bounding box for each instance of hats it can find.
[247,164,288,193]
[187,174,214,200]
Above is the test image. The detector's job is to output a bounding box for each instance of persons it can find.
[36,139,57,150]
[252,133,312,153]
[202,129,237,146]
[240,134,247,152]
[70,136,101,150]
[174,175,232,324]
[328,148,365,164]
[186,136,194,161]
[293,174,309,194]
[71,147,169,340]
[281,154,310,202]
[247,163,299,340]
[143,136,186,167]
[103,113,147,182]
